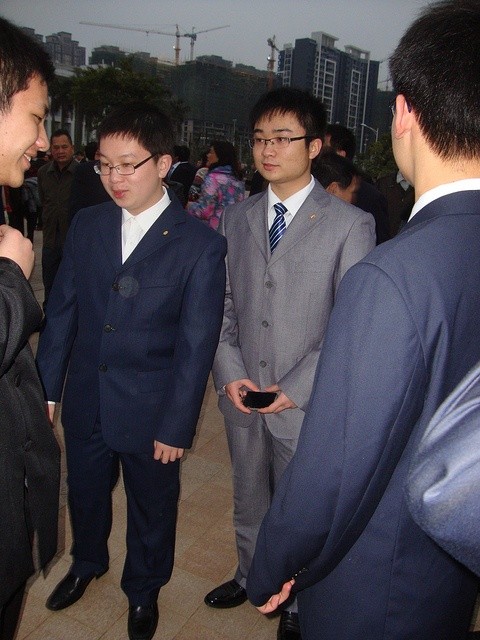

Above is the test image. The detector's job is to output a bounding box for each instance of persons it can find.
[0,124,415,313]
[0,17,62,640]
[245,0,480,640]
[206,89,376,640]
[404,359,480,574]
[35,101,229,640]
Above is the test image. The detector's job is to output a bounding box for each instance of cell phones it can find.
[243,391,277,409]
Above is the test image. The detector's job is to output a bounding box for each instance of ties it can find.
[269,203,288,255]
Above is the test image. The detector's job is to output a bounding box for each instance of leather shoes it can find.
[203,578,248,609]
[45,560,110,611]
[277,611,301,640]
[127,599,159,640]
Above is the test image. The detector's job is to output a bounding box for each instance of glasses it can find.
[251,132,317,148]
[92,151,164,176]
[390,94,412,114]
